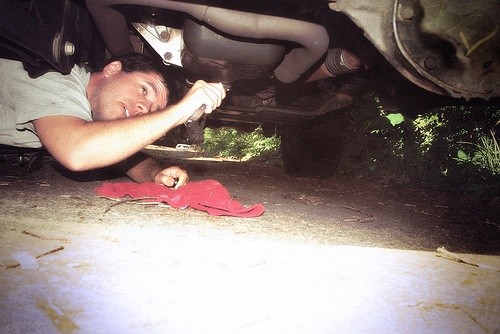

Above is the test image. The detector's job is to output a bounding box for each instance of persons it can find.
[1,50,229,193]
